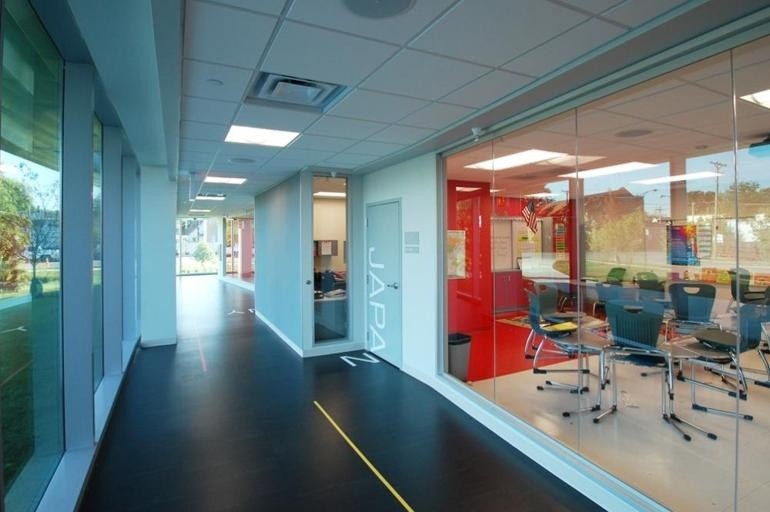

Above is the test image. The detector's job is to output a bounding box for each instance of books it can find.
[324,288,347,299]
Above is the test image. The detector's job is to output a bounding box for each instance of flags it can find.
[521,200,538,234]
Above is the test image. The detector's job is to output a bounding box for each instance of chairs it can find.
[522,267,769,445]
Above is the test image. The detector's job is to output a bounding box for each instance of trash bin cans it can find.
[448,333,471,382]
